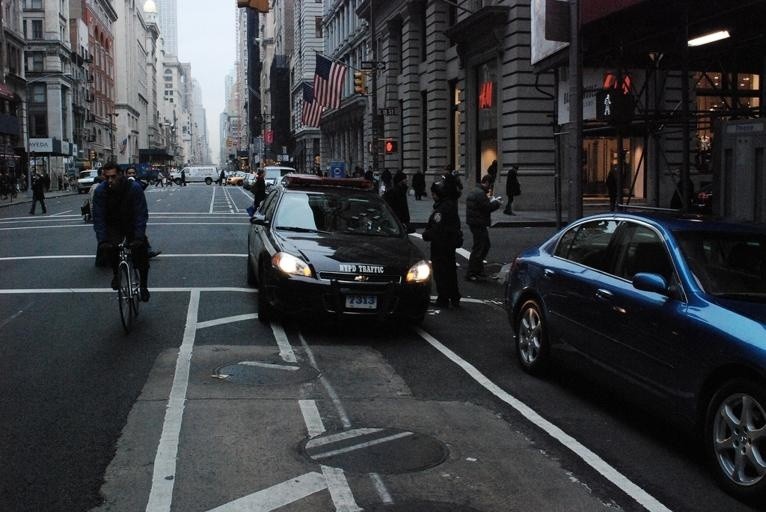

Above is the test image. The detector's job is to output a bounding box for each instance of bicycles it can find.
[111,237,142,332]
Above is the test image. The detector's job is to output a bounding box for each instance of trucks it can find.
[170,166,221,185]
[119,162,159,185]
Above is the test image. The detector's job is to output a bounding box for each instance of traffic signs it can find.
[360,61,386,70]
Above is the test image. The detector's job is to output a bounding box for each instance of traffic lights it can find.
[594,89,621,121]
[384,139,397,154]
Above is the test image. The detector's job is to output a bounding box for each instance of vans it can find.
[262,166,297,195]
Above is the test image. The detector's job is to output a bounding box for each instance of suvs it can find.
[246,172,433,335]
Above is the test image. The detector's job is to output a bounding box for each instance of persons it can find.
[180,169,188,188]
[86,160,173,301]
[218,169,226,185]
[251,170,266,211]
[0,168,78,215]
[313,157,522,307]
[603,154,630,210]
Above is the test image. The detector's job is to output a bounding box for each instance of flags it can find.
[311,53,347,110]
[301,82,323,128]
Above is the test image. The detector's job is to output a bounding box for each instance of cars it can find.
[223,170,255,189]
[503,207,766,493]
[74,169,98,194]
[687,183,712,212]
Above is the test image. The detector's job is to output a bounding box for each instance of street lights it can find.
[106,112,120,163]
[253,105,276,164]
[24,71,72,197]
